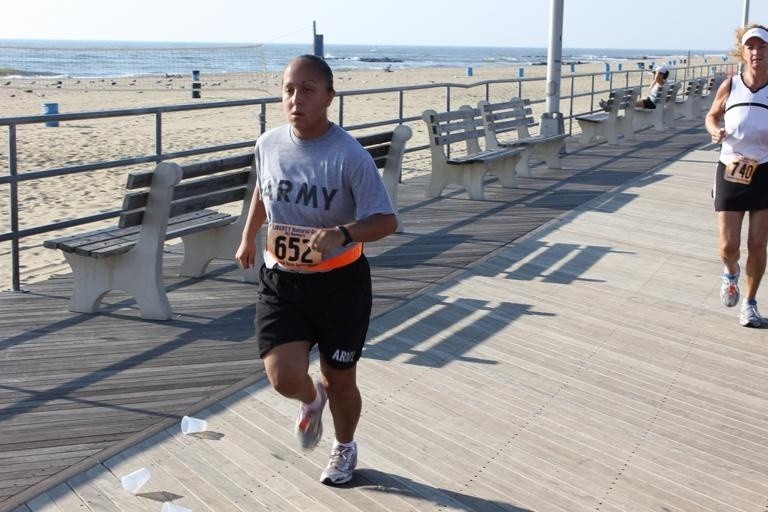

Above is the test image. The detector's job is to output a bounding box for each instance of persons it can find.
[600,65,671,112]
[704,23,768,328]
[237,53,404,486]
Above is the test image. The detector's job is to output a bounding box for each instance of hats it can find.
[741,27,768,46]
[651,66,666,74]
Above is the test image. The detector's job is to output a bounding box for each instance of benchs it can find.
[40,123,411,323]
[424,97,573,201]
[576,65,731,144]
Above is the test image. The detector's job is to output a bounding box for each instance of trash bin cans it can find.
[571,63,575,72]
[192,83,201,99]
[192,70,200,81]
[637,62,644,69]
[468,67,473,76]
[618,63,621,70]
[680,59,687,63]
[668,60,676,64]
[44,102,59,127]
[705,59,708,63]
[520,68,524,77]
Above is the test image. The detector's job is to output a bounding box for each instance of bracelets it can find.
[335,225,353,246]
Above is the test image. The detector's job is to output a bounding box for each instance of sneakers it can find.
[320,440,358,486]
[737,297,762,328]
[720,262,740,308]
[297,382,330,450]
[598,99,608,112]
